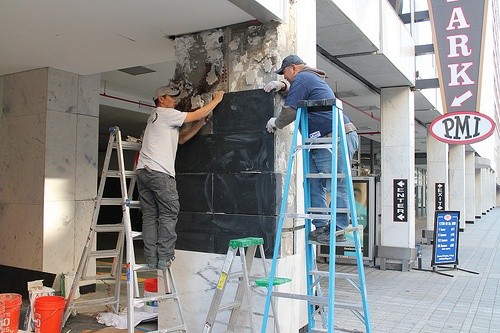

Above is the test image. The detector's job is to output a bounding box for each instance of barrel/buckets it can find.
[33,296,65,333]
[144,278,158,313]
[0,293,22,333]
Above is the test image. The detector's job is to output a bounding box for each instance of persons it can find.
[137,85,226,270]
[264,54,361,242]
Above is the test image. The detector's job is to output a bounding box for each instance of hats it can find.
[273,55,305,75]
[152,86,180,101]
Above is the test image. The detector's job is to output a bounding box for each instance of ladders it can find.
[261,100,371,333]
[202,237,279,333]
[61,127,187,333]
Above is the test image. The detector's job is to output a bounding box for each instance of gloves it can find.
[264,80,286,94]
[265,117,277,134]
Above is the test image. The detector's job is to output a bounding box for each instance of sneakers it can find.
[148,260,172,270]
[307,225,348,244]
